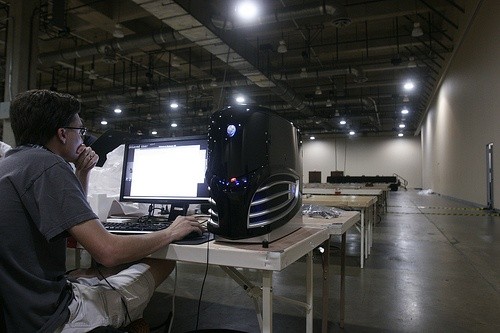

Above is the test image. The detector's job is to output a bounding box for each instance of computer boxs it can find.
[205,105,304,243]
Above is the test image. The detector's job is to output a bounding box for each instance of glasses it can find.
[64,127,88,136]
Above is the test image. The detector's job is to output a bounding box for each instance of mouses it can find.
[182,228,203,241]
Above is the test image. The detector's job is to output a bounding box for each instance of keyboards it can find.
[101,221,172,234]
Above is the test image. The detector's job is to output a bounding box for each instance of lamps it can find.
[113,0,125,38]
[411,0,424,38]
[315,72,322,94]
[278,32,288,53]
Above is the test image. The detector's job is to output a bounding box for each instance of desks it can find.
[65,184,391,333]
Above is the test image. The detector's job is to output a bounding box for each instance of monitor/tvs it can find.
[120,138,211,204]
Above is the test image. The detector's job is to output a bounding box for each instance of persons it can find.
[0,89,205,333]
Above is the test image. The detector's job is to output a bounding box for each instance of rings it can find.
[87,153,94,159]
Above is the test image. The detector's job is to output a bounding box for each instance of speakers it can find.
[89,129,129,168]
